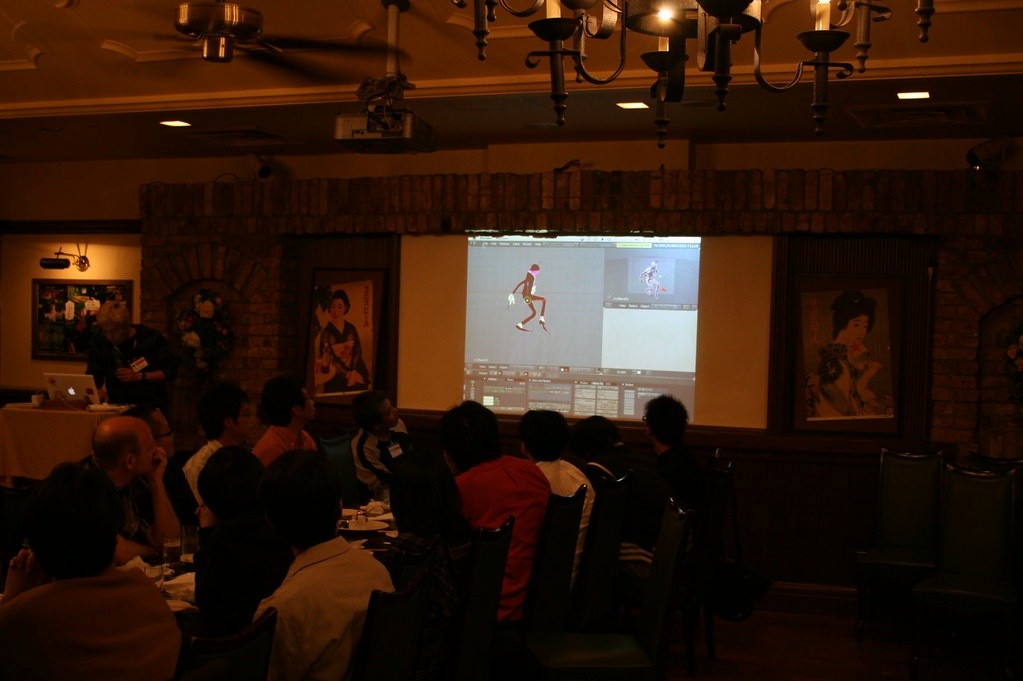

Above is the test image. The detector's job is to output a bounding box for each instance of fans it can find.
[88,0,414,88]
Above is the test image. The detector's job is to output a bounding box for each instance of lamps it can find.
[55,241,90,271]
[447,0,935,136]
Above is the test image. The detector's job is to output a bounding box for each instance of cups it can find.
[32,394,44,406]
[162,536,182,575]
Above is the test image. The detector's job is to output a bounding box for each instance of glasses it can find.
[153,427,175,441]
[231,411,254,418]
[642,415,649,423]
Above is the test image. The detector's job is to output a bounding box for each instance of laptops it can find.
[43,372,100,406]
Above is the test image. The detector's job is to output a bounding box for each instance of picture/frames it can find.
[30,278,135,360]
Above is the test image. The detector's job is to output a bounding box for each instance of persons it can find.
[181,373,408,681]
[0,414,186,681]
[413,394,728,657]
[83,300,178,424]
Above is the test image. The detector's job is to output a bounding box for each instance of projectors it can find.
[335,110,436,154]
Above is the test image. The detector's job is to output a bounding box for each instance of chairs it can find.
[165,444,733,681]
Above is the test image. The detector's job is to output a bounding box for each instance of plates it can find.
[336,509,389,531]
[120,561,195,611]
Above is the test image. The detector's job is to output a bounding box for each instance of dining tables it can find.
[155,505,396,638]
[0,403,133,488]
